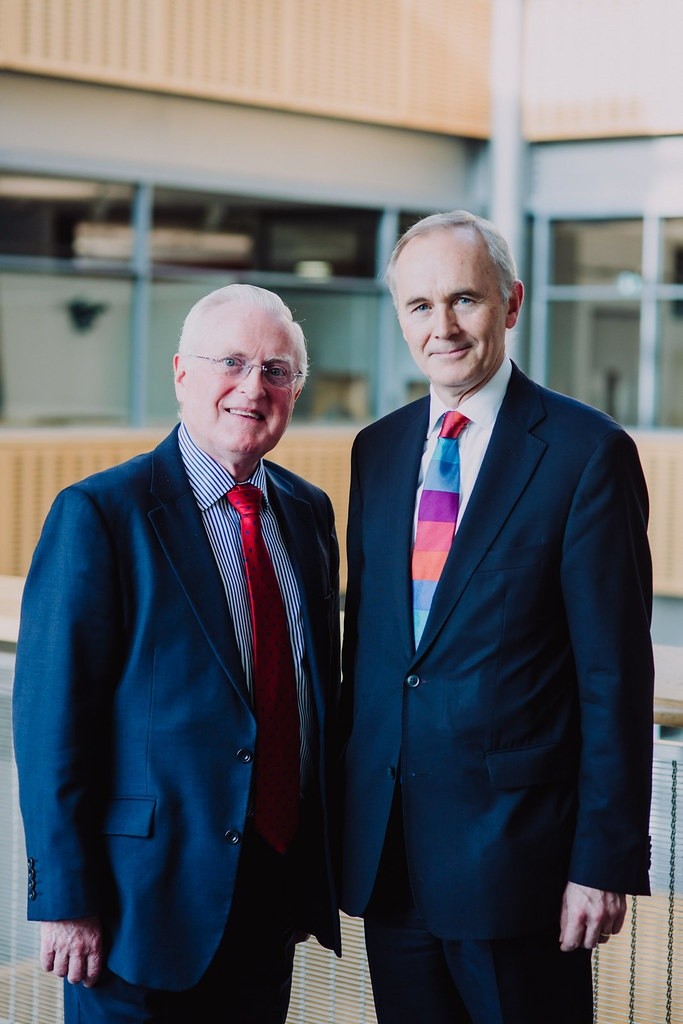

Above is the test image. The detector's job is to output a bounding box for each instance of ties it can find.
[411,412,472,650]
[227,482,302,853]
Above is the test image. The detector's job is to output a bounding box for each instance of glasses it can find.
[188,352,306,387]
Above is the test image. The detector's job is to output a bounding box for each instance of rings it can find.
[600,933,610,936]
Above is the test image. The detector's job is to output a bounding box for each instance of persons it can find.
[12,284,341,1024]
[341,211,654,1024]
